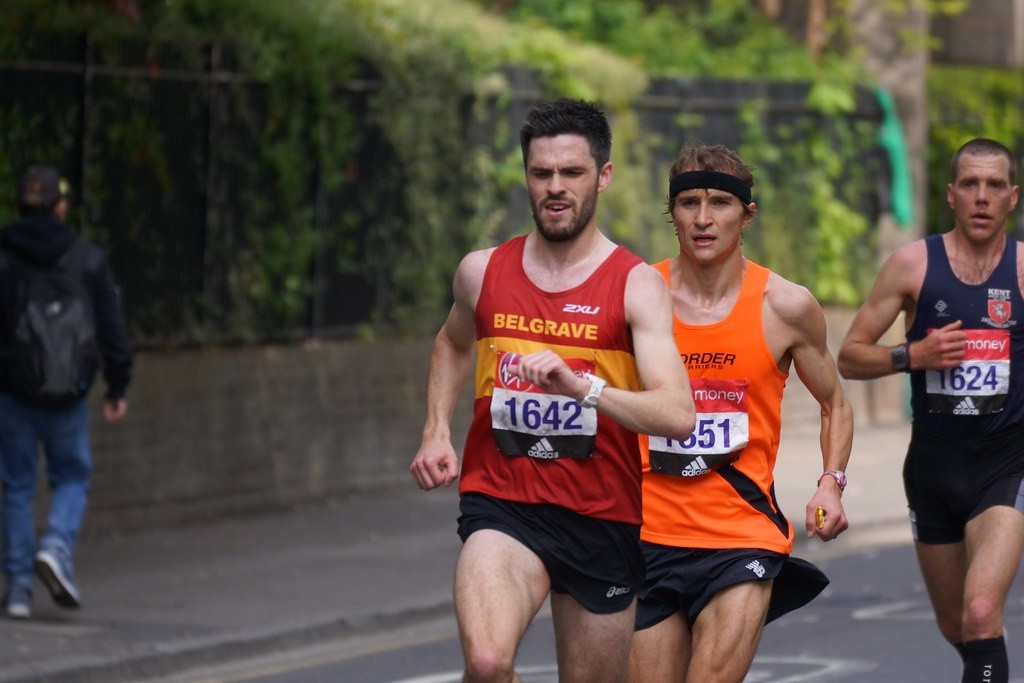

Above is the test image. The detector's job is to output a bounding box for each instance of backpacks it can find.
[0,237,102,400]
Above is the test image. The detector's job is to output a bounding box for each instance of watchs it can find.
[892,344,910,372]
[577,373,606,408]
[818,470,847,490]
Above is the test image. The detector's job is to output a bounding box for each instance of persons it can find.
[839,138,1024,683]
[412,99,696,683]
[628,144,854,683]
[0,163,132,618]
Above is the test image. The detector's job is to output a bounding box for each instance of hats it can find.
[17,165,70,212]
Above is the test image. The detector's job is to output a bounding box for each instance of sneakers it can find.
[1,591,30,618]
[34,545,80,608]
